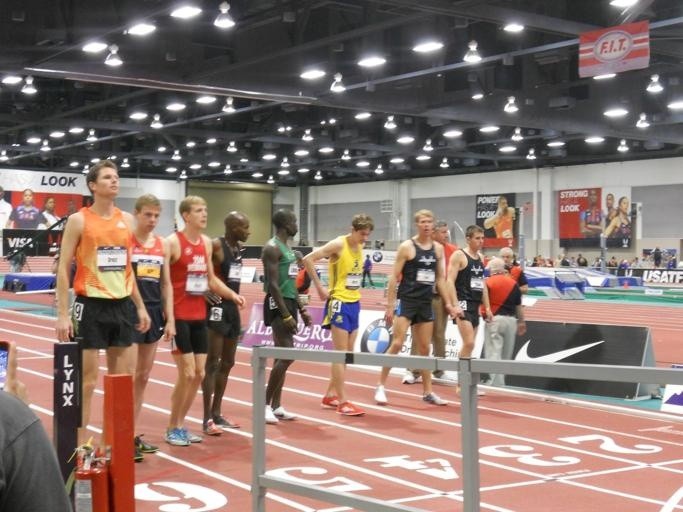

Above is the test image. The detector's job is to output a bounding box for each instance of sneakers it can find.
[321,396,340,410]
[336,401,366,416]
[423,391,449,406]
[374,384,388,404]
[264,405,278,424]
[402,369,487,397]
[272,407,297,420]
[133,435,157,461]
[165,427,203,446]
[202,414,240,436]
[76,447,108,481]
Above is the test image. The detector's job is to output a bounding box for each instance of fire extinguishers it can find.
[74,444,111,512]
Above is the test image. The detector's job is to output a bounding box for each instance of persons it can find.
[0,186,78,228]
[123,194,175,460]
[202,210,251,437]
[579,190,602,239]
[54,161,150,465]
[363,197,527,405]
[164,196,247,446]
[0,384,72,512]
[604,194,616,228]
[532,247,678,274]
[303,213,374,416]
[260,207,320,424]
[602,196,631,239]
